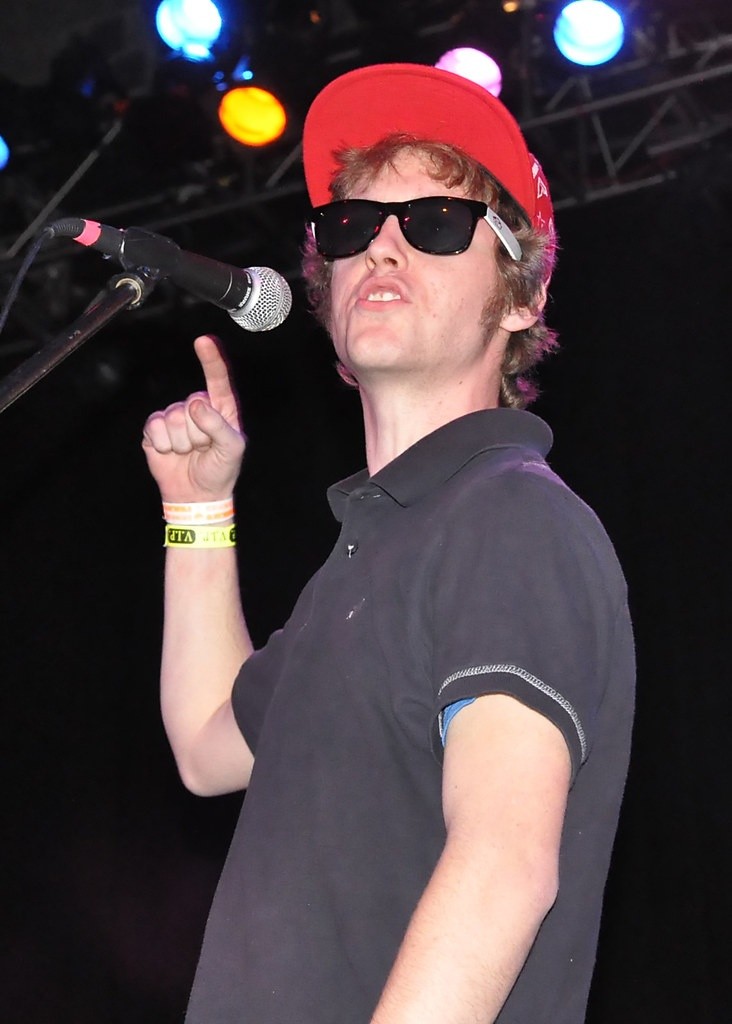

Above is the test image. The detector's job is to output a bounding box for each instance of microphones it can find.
[50,214,292,333]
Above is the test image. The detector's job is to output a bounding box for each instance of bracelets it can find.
[161,492,235,525]
[162,522,237,551]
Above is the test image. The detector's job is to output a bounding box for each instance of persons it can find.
[141,60,639,1024]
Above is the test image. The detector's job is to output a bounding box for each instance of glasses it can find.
[308,196,523,265]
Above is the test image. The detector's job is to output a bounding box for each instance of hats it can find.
[300,62,558,287]
